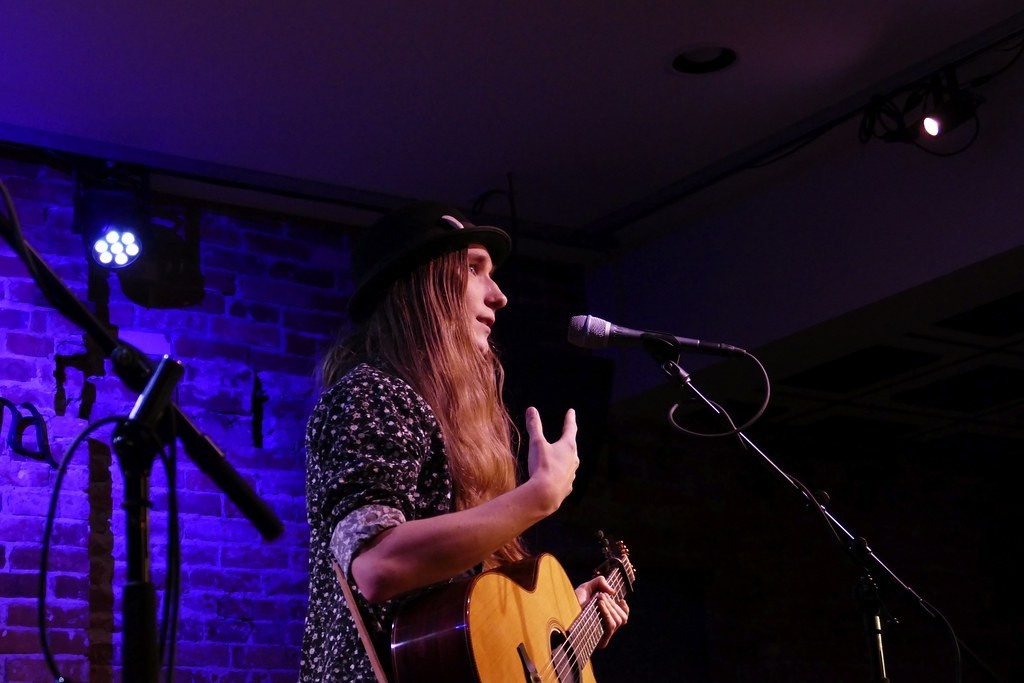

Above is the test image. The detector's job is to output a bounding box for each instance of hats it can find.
[345,200,511,318]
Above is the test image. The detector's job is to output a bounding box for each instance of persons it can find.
[296,209,630,683]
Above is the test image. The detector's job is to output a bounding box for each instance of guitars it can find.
[388,529,639,683]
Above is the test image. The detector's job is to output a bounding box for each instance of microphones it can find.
[568,314,747,357]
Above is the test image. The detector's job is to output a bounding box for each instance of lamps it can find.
[78,182,145,270]
